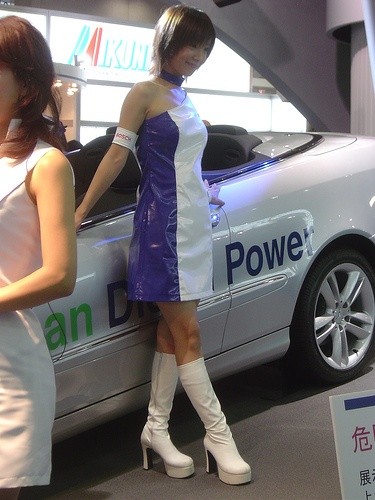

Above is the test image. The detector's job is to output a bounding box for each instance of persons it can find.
[0,15,78,500]
[75,4,253,485]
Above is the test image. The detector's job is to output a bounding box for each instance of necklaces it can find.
[158,69,185,87]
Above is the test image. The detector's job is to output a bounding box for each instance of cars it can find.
[0,118,375,447]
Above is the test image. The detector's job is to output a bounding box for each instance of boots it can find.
[142,352,194,478]
[176,357,251,485]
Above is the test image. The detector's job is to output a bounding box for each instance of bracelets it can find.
[111,126,140,152]
